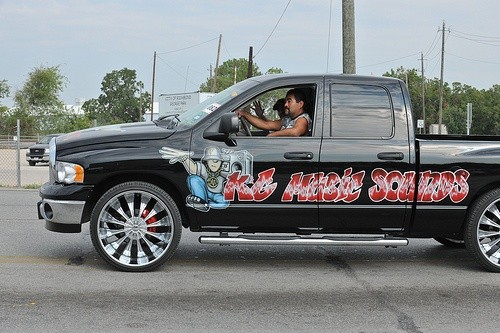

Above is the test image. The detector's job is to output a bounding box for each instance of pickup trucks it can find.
[37,73,496,273]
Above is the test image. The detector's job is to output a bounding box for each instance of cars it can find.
[26,132,63,167]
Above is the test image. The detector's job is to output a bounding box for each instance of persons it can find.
[234,88,313,137]
[249,97,286,123]
